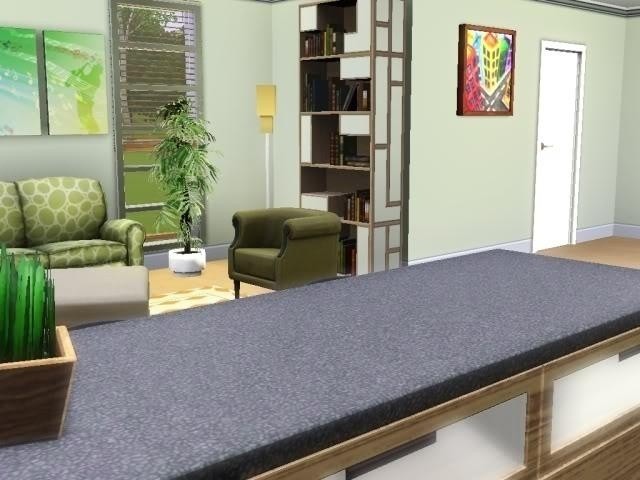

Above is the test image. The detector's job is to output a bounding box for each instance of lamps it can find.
[255,84,276,208]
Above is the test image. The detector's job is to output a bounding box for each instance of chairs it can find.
[227,206,342,298]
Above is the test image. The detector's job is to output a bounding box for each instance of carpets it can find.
[149,283,254,317]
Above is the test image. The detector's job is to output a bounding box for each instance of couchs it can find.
[0,176,147,269]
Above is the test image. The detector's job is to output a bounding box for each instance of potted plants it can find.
[146,93,226,277]
[0,243,79,445]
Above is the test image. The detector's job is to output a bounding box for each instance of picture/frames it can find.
[456,23,517,116]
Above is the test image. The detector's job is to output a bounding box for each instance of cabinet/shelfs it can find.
[298,0,413,278]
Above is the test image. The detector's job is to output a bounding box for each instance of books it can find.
[329,128,370,166]
[303,73,371,112]
[338,235,357,275]
[302,24,346,57]
[345,188,370,224]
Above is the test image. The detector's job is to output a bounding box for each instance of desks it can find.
[0,247,640,480]
